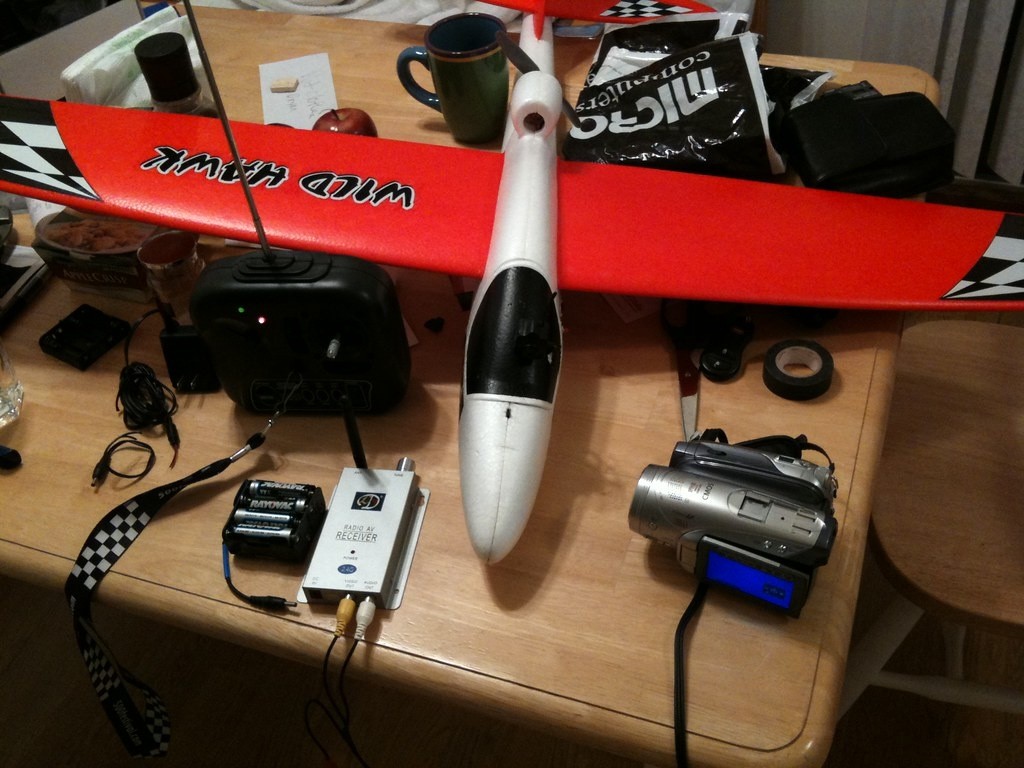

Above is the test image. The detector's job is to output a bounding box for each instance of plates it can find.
[37,215,145,254]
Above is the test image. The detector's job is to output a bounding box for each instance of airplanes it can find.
[0,2,1024,571]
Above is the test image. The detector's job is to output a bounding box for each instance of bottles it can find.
[138,230,206,322]
[134,32,220,118]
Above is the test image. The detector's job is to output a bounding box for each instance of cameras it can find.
[628,440,838,620]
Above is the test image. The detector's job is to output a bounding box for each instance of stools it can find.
[834,322,1024,727]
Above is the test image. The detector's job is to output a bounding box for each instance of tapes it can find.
[762,339,835,402]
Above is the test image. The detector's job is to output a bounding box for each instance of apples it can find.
[311,107,378,137]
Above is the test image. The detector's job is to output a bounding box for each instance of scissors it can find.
[661,291,712,447]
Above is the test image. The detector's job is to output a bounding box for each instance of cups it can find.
[397,12,509,142]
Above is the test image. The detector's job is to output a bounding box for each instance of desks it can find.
[0,0,941,768]
